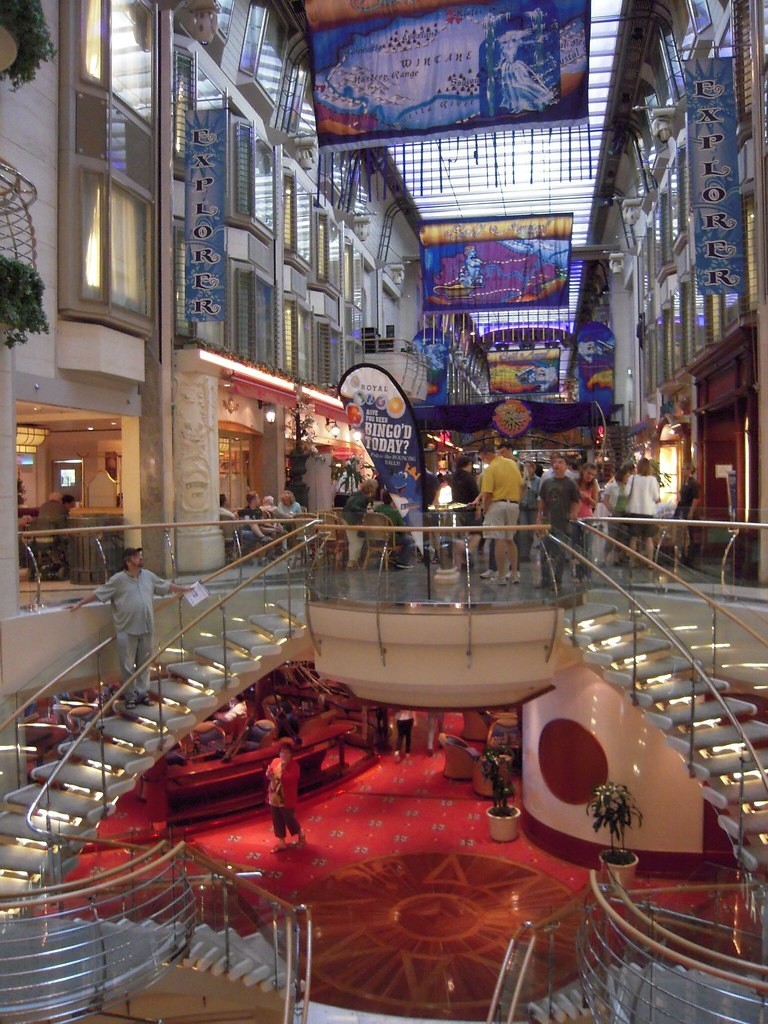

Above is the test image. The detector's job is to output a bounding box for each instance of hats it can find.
[123,547,143,558]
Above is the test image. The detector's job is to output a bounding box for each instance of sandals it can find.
[125,699,136,709]
[137,696,155,706]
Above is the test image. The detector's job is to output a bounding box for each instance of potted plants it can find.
[0,254,49,349]
[481,747,521,841]
[586,784,642,888]
[0,0,58,92]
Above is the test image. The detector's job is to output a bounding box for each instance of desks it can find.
[473,753,512,796]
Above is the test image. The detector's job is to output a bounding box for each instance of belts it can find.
[496,499,518,504]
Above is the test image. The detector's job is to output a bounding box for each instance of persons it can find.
[220,441,702,589]
[17,491,123,581]
[375,707,445,763]
[62,547,194,710]
[165,693,299,767]
[265,742,306,853]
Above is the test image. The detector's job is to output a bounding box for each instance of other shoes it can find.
[270,844,286,853]
[296,836,306,847]
[644,561,654,571]
[497,576,507,585]
[535,579,548,589]
[396,563,413,568]
[480,570,495,579]
[510,575,521,584]
[552,581,560,591]
[431,556,438,564]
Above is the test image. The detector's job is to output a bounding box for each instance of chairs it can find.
[217,506,402,577]
[155,693,297,767]
[26,519,65,581]
[439,709,519,780]
[24,685,117,764]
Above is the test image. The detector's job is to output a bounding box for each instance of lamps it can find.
[326,417,340,438]
[609,254,625,274]
[349,424,361,442]
[622,199,643,226]
[257,399,276,424]
[16,424,50,453]
[651,107,676,144]
